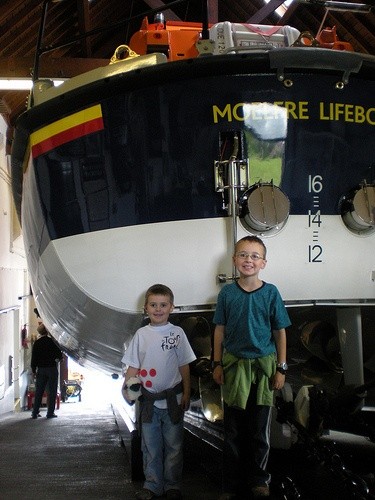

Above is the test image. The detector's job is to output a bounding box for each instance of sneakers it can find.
[135,488,155,500]
[217,493,236,500]
[251,486,269,498]
[165,489,181,500]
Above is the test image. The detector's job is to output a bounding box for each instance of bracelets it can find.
[276,367,288,375]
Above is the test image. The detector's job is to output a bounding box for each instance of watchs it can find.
[276,362,289,371]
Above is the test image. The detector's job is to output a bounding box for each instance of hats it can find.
[37,324,46,335]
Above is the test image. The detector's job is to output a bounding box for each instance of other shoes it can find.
[31,413,41,417]
[47,413,57,418]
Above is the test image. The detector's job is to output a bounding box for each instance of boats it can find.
[7,15,374,498]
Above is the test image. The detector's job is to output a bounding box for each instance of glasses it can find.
[238,251,264,261]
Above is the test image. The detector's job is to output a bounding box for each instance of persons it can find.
[31,324,64,419]
[210,234,293,500]
[121,284,198,500]
